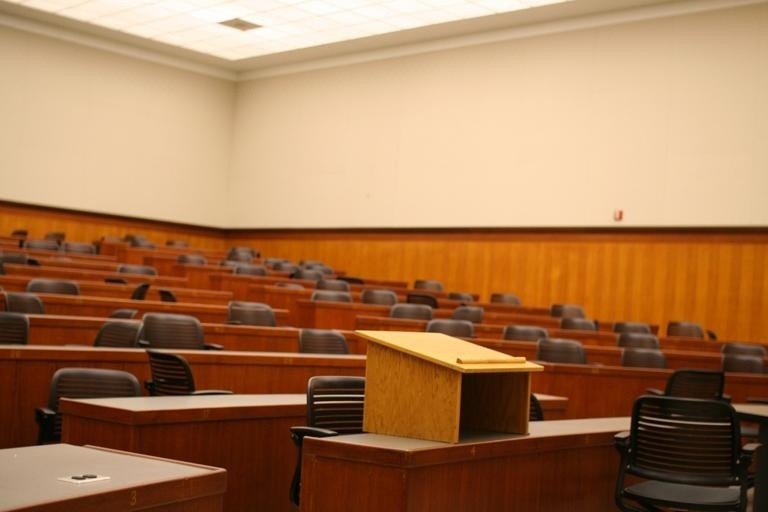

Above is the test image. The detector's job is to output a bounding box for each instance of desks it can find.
[59,391,568,510]
[0,343,586,446]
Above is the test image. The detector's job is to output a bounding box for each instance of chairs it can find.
[0,274,767,371]
[1,228,334,280]
[613,394,763,510]
[647,370,730,400]
[33,368,141,447]
[145,351,234,396]
[290,376,365,506]
